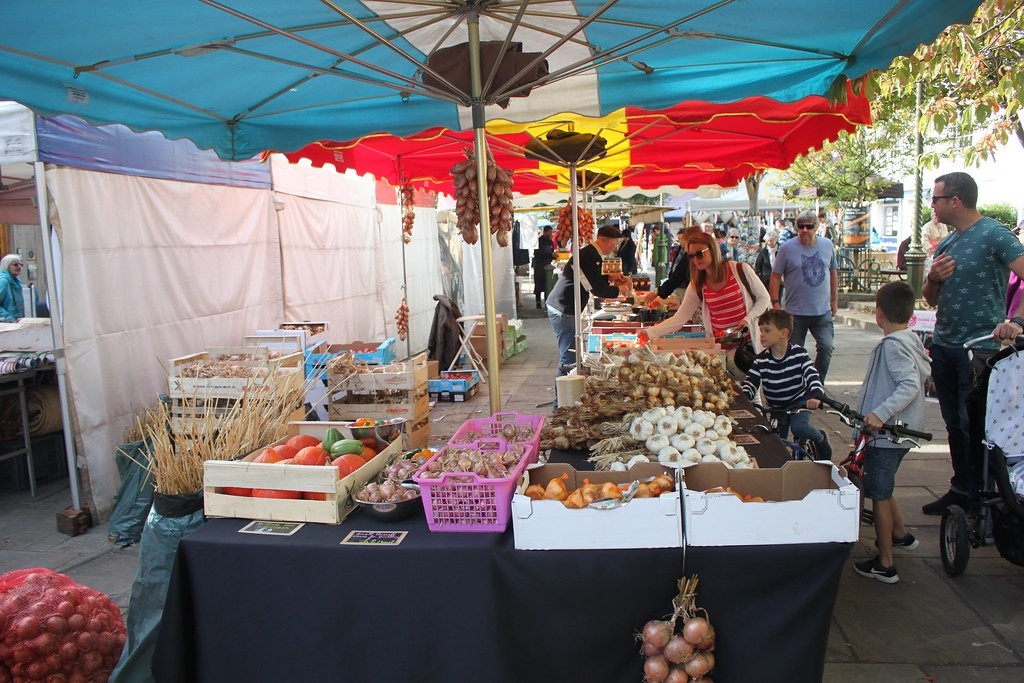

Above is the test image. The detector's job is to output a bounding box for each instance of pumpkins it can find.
[224,434,377,501]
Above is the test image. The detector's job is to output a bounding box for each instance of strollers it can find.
[941,331,1024,578]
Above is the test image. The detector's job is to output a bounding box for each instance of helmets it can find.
[726,348,755,381]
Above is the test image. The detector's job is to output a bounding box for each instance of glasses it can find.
[797,222,816,229]
[687,246,711,261]
[731,236,739,239]
[11,263,24,267]
[931,196,961,205]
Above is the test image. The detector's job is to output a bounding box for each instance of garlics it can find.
[548,350,735,451]
[610,406,752,471]
[524,473,673,509]
[705,488,764,502]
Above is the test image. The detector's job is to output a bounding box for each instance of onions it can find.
[557,204,595,247]
[638,616,716,683]
[358,423,533,525]
[394,305,410,341]
[401,183,416,244]
[449,161,515,248]
[0,571,127,683]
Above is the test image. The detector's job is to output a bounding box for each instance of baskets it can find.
[412,438,534,533]
[447,412,546,463]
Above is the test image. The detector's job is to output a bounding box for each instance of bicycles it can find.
[812,392,932,526]
[752,402,823,461]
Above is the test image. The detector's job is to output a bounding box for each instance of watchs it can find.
[1009,316,1024,333]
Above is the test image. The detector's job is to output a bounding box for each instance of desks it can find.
[151,499,854,683]
[546,371,794,468]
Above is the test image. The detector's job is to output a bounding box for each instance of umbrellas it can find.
[257,69,872,378]
[817,160,904,289]
[408,165,766,333]
[0,0,989,430]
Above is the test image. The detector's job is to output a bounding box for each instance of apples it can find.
[438,373,470,381]
[350,348,377,353]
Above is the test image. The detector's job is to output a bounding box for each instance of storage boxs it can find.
[587,260,727,370]
[680,461,861,546]
[169,312,528,526]
[0,433,67,494]
[510,459,685,551]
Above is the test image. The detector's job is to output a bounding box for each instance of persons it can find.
[0,253,25,321]
[819,213,837,245]
[642,225,704,332]
[531,226,559,309]
[897,235,911,271]
[921,173,1023,514]
[770,210,839,410]
[536,209,881,302]
[853,281,933,583]
[921,208,949,309]
[637,232,774,417]
[670,228,691,304]
[1000,220,1024,351]
[740,308,833,459]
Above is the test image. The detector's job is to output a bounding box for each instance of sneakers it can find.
[875,533,919,551]
[854,554,899,584]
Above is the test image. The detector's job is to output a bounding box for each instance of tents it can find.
[686,170,819,226]
[0,100,520,536]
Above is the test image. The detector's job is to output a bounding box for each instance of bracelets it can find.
[927,275,940,284]
[771,300,779,305]
[553,253,554,255]
[654,292,657,295]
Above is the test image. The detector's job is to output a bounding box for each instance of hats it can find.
[598,226,623,238]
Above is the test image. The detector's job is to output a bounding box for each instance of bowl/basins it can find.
[395,446,444,464]
[345,417,408,442]
[352,483,421,520]
[713,326,746,345]
[379,460,427,483]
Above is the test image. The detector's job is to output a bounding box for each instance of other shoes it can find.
[814,429,832,460]
[922,489,968,515]
[536,302,542,309]
